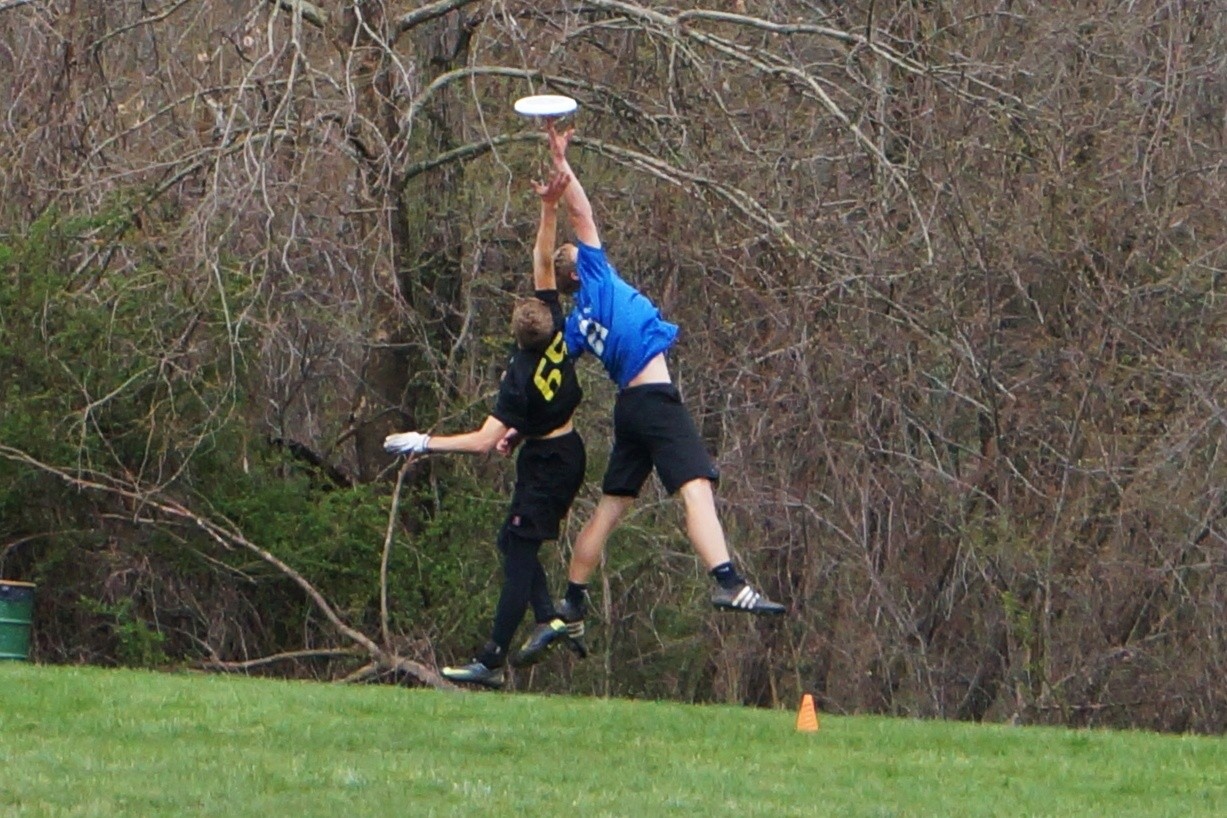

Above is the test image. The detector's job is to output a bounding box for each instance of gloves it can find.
[384,431,429,453]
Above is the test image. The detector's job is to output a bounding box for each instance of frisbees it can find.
[512,94,578,116]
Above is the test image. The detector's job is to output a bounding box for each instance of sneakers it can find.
[440,660,509,689]
[712,581,786,614]
[551,618,588,659]
[510,621,564,665]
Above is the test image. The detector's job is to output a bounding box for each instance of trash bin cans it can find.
[0,580,38,664]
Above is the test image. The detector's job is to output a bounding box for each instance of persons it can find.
[378,170,590,686]
[528,115,791,647]
